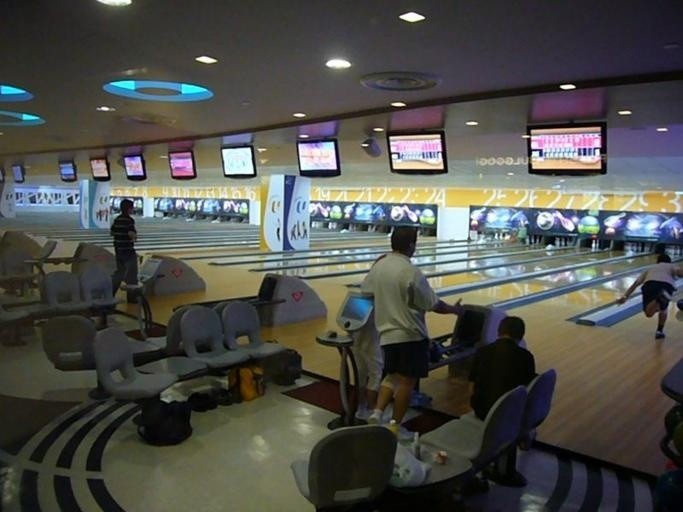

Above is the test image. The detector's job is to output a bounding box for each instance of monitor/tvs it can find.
[138,259,160,277]
[123,154,146,180]
[221,145,256,177]
[527,121,607,175]
[386,130,447,175]
[0,168,4,182]
[90,158,110,181]
[59,160,76,181]
[339,295,374,323]
[168,150,196,179]
[296,139,340,177]
[12,164,24,182]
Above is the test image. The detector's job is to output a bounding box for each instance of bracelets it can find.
[622,295,627,300]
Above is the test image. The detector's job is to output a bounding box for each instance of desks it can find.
[124,336,161,355]
[135,358,211,380]
[9,304,51,335]
[397,442,473,486]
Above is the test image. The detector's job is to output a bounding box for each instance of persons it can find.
[361,225,462,426]
[110,199,139,304]
[615,254,683,339]
[468,316,537,487]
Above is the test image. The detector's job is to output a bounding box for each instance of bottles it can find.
[252,368,266,397]
[57,351,83,361]
[410,391,433,407]
[410,431,421,459]
[387,419,399,439]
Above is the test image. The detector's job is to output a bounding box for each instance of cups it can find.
[435,451,448,465]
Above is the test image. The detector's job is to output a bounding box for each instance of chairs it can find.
[145,306,193,354]
[46,271,91,315]
[461,368,556,488]
[93,328,179,429]
[32,248,46,277]
[41,315,114,401]
[291,425,397,508]
[5,254,39,297]
[222,301,287,366]
[214,301,231,317]
[1,272,6,283]
[0,306,31,347]
[78,261,125,329]
[418,386,527,476]
[180,306,250,377]
